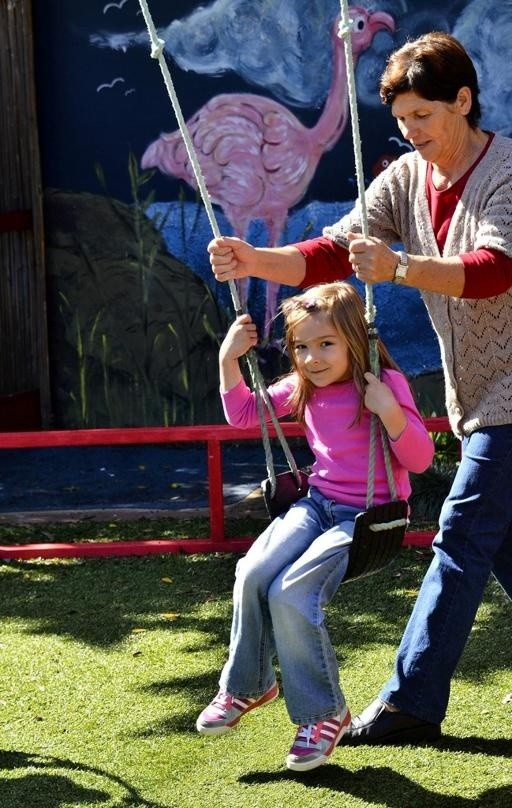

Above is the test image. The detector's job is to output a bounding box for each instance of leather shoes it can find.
[337,697,441,747]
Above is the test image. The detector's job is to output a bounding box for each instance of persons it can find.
[207,31,512,744]
[194,284,436,772]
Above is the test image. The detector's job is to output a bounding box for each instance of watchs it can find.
[393,248,409,285]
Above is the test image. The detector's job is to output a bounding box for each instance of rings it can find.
[355,264,362,274]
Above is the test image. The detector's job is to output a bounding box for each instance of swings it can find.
[137,0,407,584]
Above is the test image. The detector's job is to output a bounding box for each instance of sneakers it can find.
[286,705,351,771]
[196,682,279,734]
